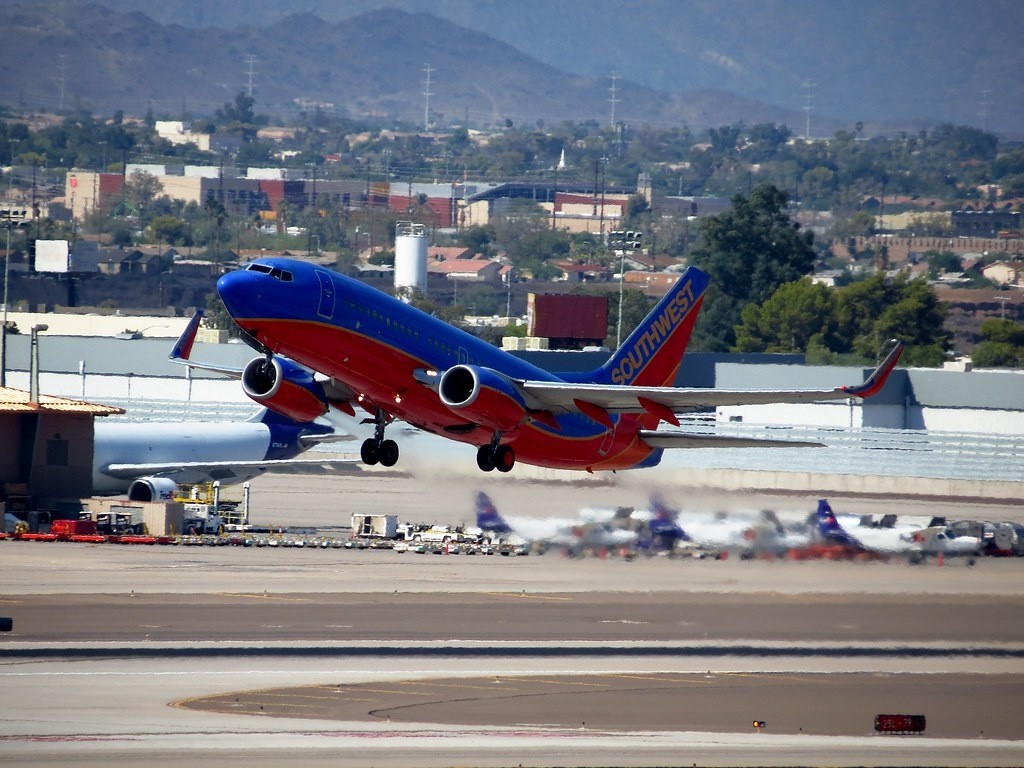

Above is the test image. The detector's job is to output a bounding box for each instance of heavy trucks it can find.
[185,503,227,536]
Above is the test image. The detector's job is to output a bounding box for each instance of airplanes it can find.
[165,256,906,473]
[816,499,983,566]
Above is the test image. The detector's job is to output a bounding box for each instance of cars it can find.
[396,522,484,543]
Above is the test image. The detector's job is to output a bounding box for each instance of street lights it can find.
[608,230,643,350]
[1,210,27,321]
